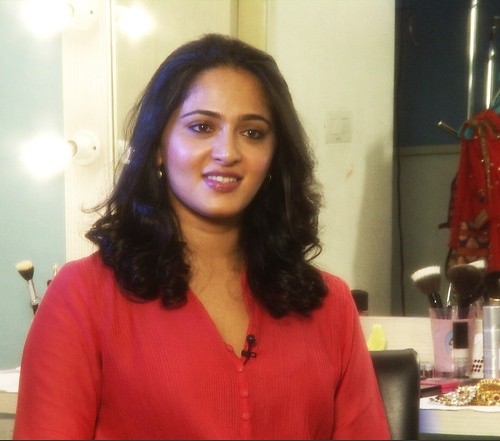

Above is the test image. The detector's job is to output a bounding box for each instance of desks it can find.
[0,364,500,441]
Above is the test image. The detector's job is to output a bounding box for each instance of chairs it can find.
[368,349,419,441]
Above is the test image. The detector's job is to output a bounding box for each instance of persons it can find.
[13,36,390,441]
